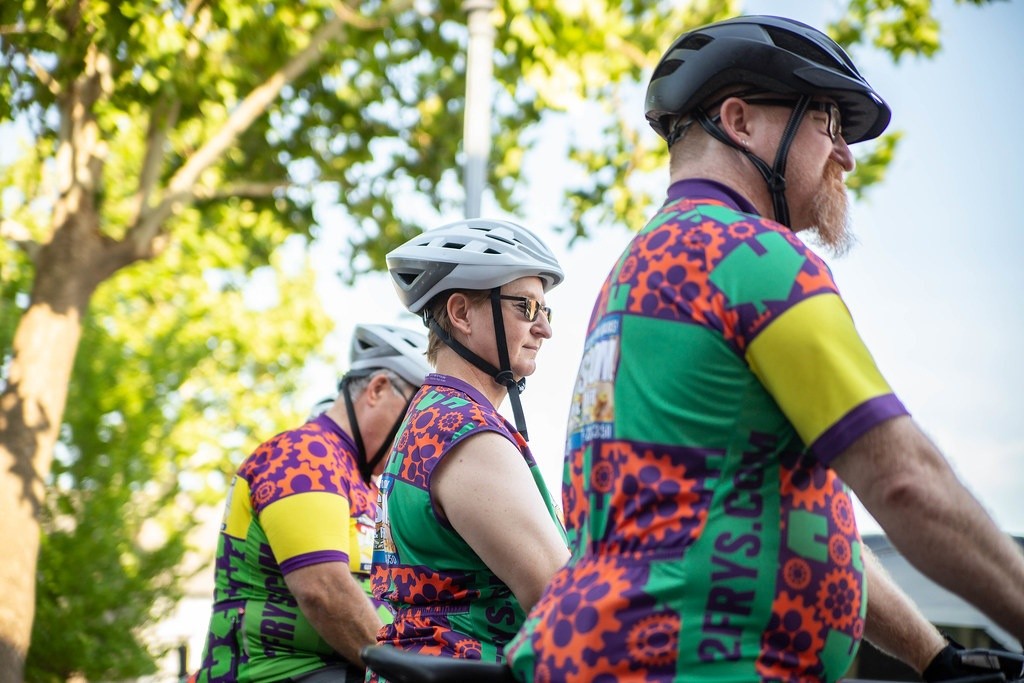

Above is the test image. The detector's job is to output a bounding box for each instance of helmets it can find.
[385,217,565,314]
[349,321,437,389]
[645,14,891,145]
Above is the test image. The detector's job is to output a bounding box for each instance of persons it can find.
[196,324,438,682]
[500,14,1023,682]
[371,217,574,682]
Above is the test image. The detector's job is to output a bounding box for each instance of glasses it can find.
[710,95,844,144]
[489,291,552,325]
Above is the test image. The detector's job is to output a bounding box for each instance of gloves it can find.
[919,634,1024,683]
